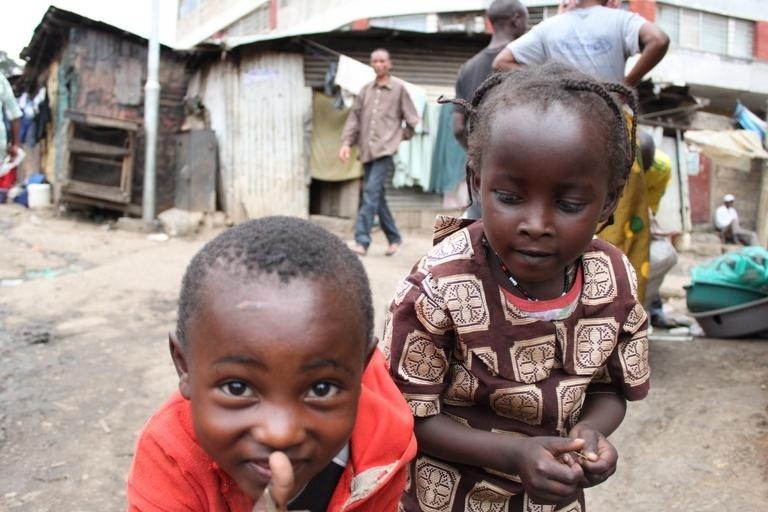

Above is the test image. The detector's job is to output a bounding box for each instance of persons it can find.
[714,194,757,246]
[449,0,529,219]
[126,215,418,512]
[0,72,22,171]
[638,131,678,329]
[379,59,650,512]
[338,48,420,256]
[491,0,670,306]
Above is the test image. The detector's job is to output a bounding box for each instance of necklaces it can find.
[494,252,570,302]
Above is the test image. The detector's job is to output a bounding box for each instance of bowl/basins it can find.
[682,281,766,312]
[688,301,766,349]
